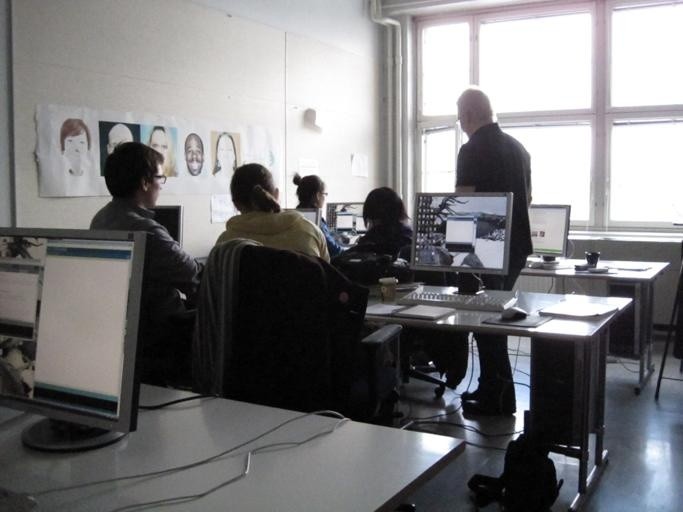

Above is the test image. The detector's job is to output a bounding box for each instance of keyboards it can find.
[401,290,519,312]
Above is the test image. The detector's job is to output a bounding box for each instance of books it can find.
[538,300,619,321]
[365,303,456,321]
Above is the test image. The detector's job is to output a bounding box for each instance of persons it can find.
[294,175,352,258]
[455,89,533,416]
[149,127,178,177]
[212,132,238,178]
[344,187,413,266]
[107,124,134,156]
[59,118,91,177]
[212,163,332,266]
[185,134,204,176]
[90,141,205,389]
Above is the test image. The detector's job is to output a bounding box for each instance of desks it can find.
[366,285,634,511]
[1,384,469,512]
[520,255,671,394]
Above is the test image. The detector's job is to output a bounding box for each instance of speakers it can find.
[530,334,606,447]
[605,280,660,360]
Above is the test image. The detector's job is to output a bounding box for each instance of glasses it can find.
[455,115,464,124]
[320,193,327,196]
[152,175,166,184]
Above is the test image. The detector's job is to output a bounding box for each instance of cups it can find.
[585,251,600,267]
[378,276,396,303]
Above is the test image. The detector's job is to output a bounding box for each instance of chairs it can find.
[203,237,403,425]
[338,242,465,397]
[139,268,209,385]
[654,259,682,401]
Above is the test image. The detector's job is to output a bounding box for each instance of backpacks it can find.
[467,434,563,511]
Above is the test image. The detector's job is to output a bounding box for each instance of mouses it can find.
[500,304,526,320]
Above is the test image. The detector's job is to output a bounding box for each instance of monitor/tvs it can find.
[0,227,147,454]
[413,190,515,292]
[324,201,377,253]
[148,204,184,250]
[277,208,322,238]
[520,203,571,269]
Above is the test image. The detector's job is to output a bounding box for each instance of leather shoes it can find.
[464,399,513,416]
[461,389,479,399]
